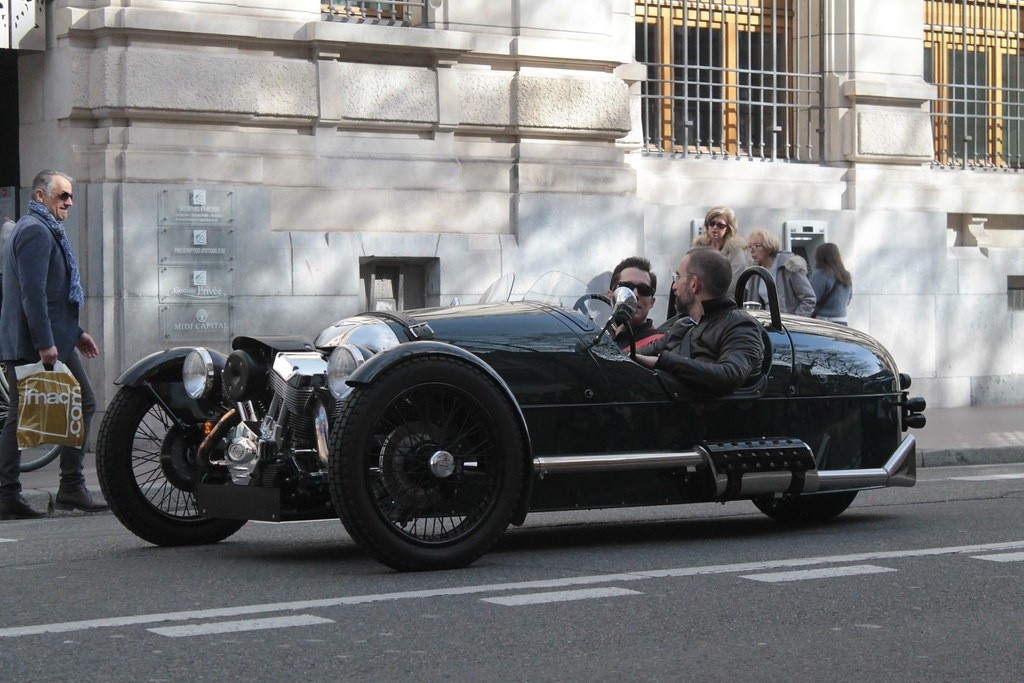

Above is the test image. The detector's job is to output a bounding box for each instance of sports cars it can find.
[93,265,927,572]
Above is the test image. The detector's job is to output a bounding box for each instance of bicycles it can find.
[0,365,61,472]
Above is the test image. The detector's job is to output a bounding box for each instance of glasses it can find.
[612,281,654,297]
[746,244,763,250]
[707,220,728,229]
[675,273,702,289]
[57,191,73,201]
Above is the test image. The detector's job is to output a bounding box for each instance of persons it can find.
[808,243,852,327]
[621,246,765,395]
[0,170,108,520]
[745,229,817,319]
[692,206,748,296]
[606,256,665,351]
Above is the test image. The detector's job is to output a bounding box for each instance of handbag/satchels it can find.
[14,359,84,450]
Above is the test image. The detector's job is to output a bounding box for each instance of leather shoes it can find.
[0,493,48,519]
[54,476,110,512]
